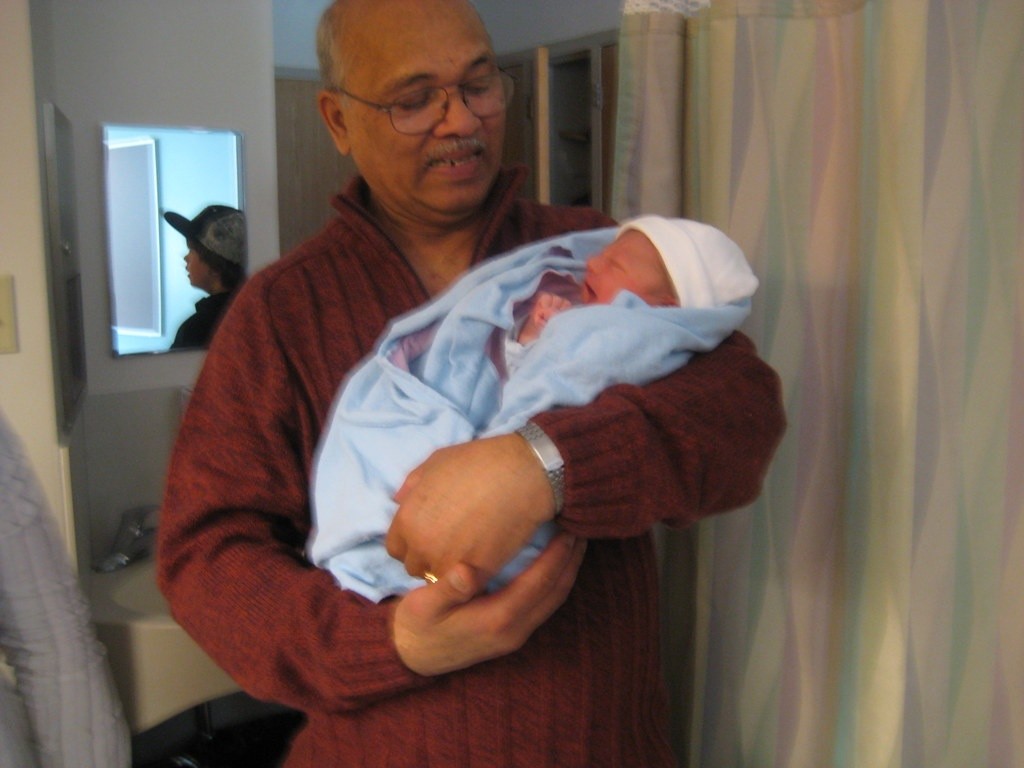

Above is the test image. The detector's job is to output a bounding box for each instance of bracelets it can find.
[515,421,566,515]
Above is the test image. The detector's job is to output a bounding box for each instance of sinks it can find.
[107,562,169,611]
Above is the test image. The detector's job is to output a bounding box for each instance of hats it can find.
[164,205,246,266]
[616,216,759,310]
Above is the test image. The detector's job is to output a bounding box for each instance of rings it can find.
[424,572,438,584]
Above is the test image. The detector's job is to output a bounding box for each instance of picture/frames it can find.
[42,102,90,424]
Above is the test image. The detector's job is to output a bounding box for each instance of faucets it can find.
[91,503,160,575]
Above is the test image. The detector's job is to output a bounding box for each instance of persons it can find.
[157,0,786,768]
[164,205,247,352]
[0,414,132,768]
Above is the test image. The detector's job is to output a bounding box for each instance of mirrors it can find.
[103,124,248,356]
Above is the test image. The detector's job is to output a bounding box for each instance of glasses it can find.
[324,65,518,136]
